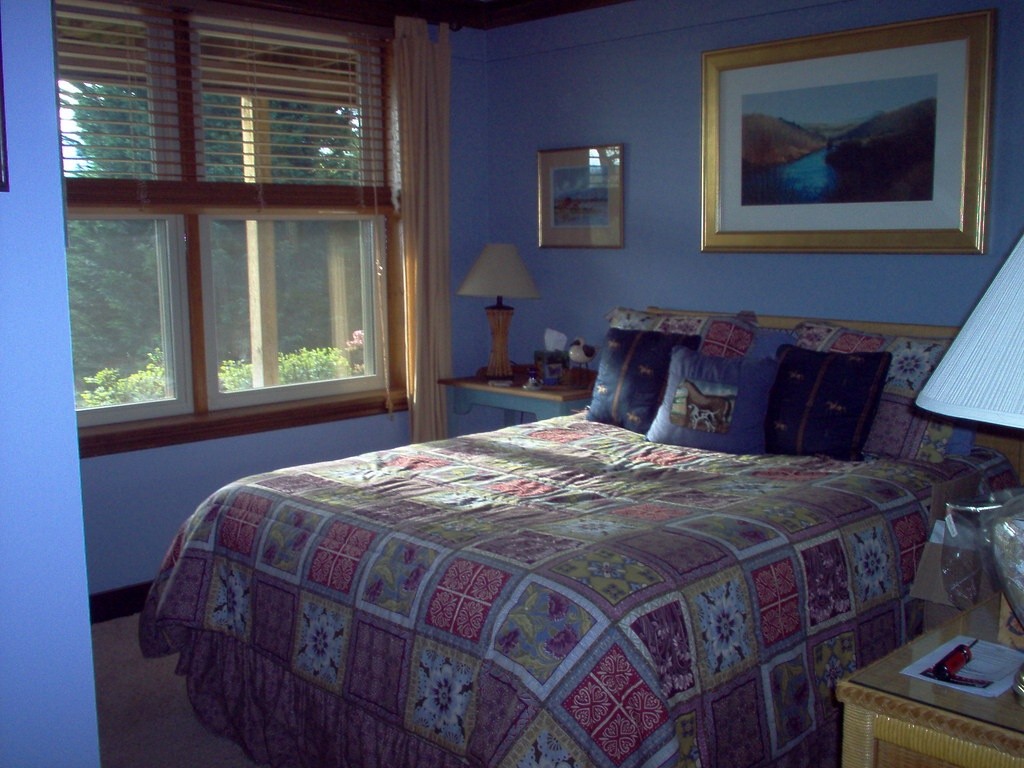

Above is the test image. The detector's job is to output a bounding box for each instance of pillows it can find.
[766,342,894,463]
[584,327,704,431]
[699,315,801,355]
[605,306,759,336]
[646,345,782,456]
[798,322,977,467]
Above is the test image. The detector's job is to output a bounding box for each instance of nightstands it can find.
[835,590,1024,768]
[437,367,593,438]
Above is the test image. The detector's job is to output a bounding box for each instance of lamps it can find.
[912,236,1024,709]
[454,243,542,381]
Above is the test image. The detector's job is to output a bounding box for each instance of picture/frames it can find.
[537,143,626,249]
[700,8,999,255]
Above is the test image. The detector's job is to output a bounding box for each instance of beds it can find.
[138,321,1024,768]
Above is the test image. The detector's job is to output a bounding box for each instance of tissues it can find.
[533,328,569,384]
